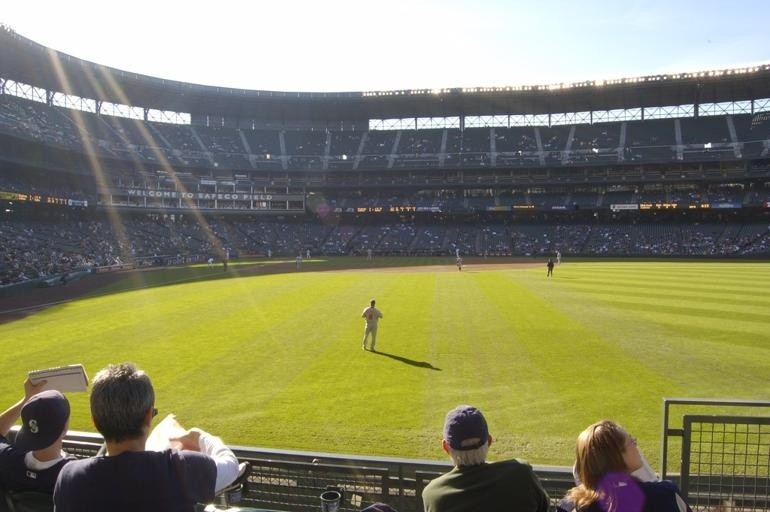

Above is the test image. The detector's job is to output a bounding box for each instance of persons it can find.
[360,297,384,352]
[53,362,239,512]
[0,374,79,512]
[552,419,693,512]
[421,403,552,512]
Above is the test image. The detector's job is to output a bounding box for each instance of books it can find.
[28,364,89,394]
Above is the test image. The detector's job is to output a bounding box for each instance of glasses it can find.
[151,407,159,419]
[620,435,639,453]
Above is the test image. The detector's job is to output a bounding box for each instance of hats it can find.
[443,405,489,451]
[15,389,71,450]
